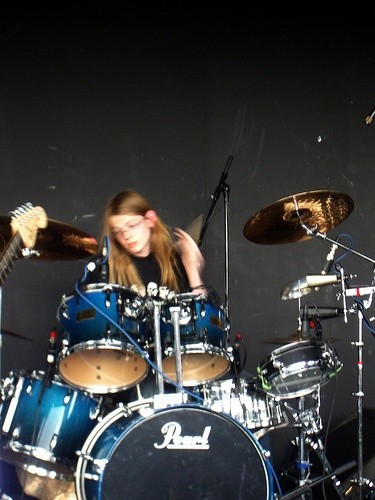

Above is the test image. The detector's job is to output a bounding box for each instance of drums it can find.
[182,374,296,434]
[148,293,233,388]
[76,395,272,500]
[1,373,115,481]
[256,342,345,399]
[54,283,150,393]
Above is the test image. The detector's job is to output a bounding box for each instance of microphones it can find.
[233,332,242,376]
[44,329,56,386]
[101,240,107,282]
[303,302,309,340]
[321,236,339,274]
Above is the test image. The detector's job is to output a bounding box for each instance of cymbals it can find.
[0,208,100,261]
[242,186,354,245]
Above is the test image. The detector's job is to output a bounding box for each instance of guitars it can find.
[0,202,48,288]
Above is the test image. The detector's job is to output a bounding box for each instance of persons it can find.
[105,189,206,299]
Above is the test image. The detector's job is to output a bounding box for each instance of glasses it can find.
[110,216,144,240]
